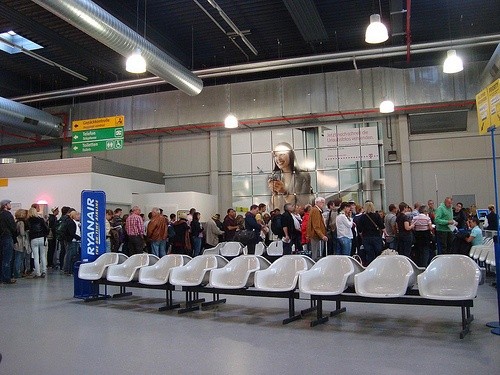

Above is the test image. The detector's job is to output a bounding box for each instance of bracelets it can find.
[282,191,289,197]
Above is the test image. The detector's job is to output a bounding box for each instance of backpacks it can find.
[271,214,282,235]
[55,217,67,240]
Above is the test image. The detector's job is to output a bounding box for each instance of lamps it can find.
[365,13,390,44]
[126,50,148,73]
[442,49,464,74]
[224,114,240,129]
[380,95,395,114]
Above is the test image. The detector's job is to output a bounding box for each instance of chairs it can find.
[78,236,496,339]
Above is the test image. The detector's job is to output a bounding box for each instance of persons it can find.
[266,142,313,214]
[0,196,497,285]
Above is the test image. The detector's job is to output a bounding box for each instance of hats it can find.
[179,213,187,218]
[272,145,292,155]
[69,208,76,213]
[51,206,60,213]
[61,206,70,216]
[0,200,12,206]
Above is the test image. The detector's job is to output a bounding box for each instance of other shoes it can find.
[3,278,16,284]
[41,272,46,278]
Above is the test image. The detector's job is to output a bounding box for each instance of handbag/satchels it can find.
[326,227,332,240]
[167,224,175,238]
[377,227,385,240]
[350,226,365,258]
[385,235,396,242]
[241,231,254,239]
[41,219,49,235]
[46,228,53,239]
